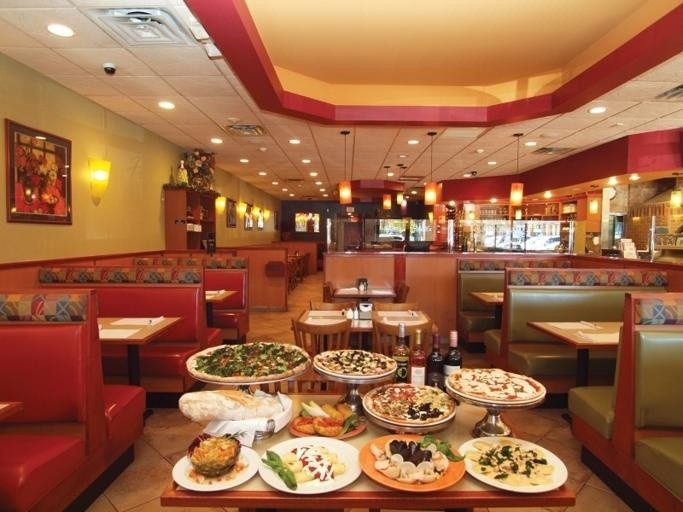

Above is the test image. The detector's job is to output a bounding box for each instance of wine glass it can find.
[185,368,309,395]
[312,367,394,414]
[437,382,548,437]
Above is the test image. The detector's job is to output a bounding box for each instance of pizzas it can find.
[365,383,455,425]
[313,349,397,376]
[185,341,313,383]
[448,368,544,402]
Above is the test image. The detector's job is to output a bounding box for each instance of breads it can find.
[178,389,283,423]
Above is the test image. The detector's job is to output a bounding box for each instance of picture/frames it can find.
[5,117,73,226]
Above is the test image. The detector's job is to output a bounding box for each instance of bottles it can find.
[176,160,188,185]
[393,322,462,406]
[172,447,259,493]
[479,206,508,220]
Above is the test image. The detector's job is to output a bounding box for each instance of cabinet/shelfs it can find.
[162,181,221,252]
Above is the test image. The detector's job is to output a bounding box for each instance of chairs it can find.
[292,278,427,390]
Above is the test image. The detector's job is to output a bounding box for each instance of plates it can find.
[360,434,466,492]
[289,421,368,439]
[260,437,362,495]
[458,436,566,493]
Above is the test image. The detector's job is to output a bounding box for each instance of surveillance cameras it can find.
[103,64,117,75]
[471,171,477,176]
